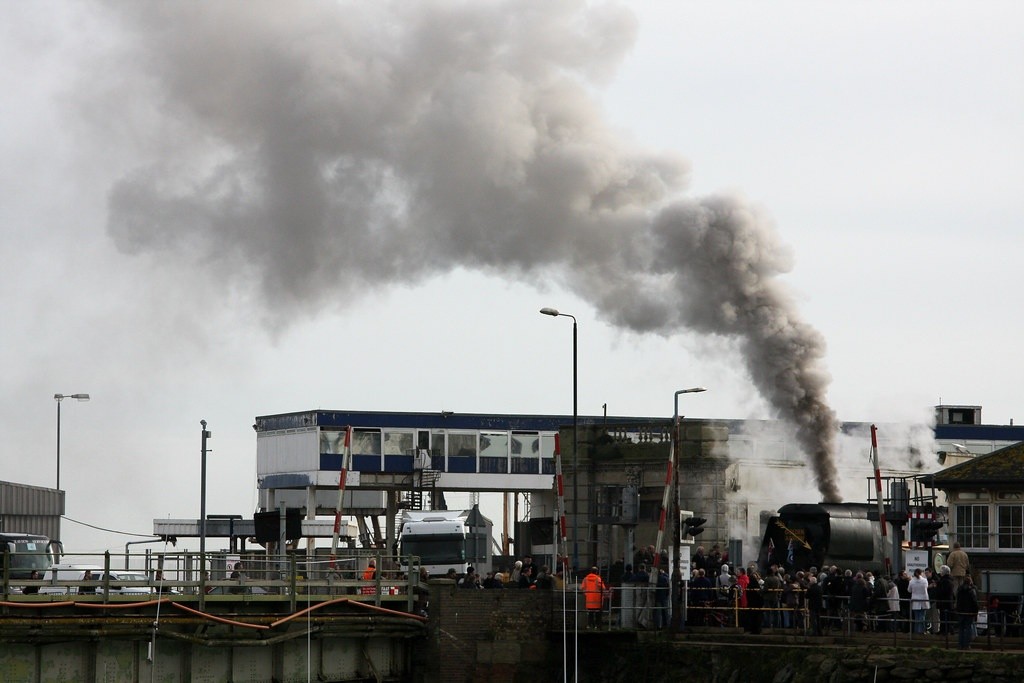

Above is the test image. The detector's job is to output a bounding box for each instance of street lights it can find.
[197,419,214,615]
[539,306,581,573]
[673,386,708,643]
[53,393,91,493]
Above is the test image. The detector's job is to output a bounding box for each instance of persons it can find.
[615,544,670,629]
[956,575,977,650]
[510,554,563,590]
[155,571,171,592]
[363,560,388,579]
[78,571,96,595]
[934,564,955,635]
[762,563,940,634]
[205,572,212,594]
[229,563,249,595]
[581,567,605,628]
[947,542,969,596]
[30,570,39,593]
[447,567,482,589]
[334,564,343,580]
[480,566,509,589]
[688,544,764,635]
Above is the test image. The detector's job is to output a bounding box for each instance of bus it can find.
[0,532,65,595]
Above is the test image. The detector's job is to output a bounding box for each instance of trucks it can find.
[36,564,157,598]
[395,508,500,585]
